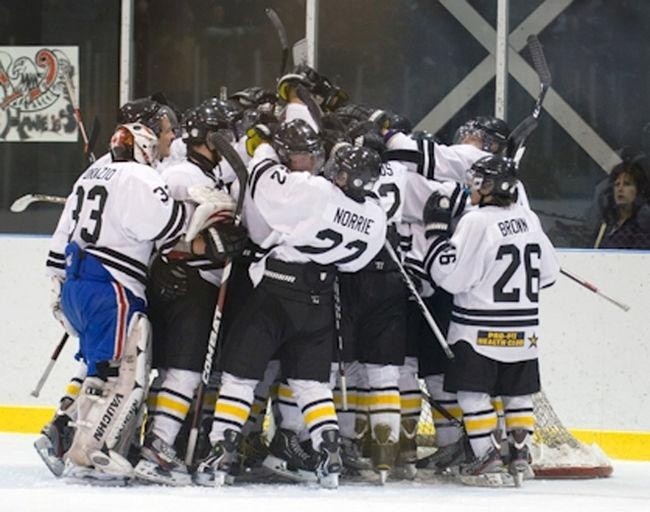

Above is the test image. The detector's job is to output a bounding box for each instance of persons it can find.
[592,159,649,248]
[32,67,561,487]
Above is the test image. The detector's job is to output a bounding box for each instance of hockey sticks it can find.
[10,193,68,213]
[415,388,469,469]
[512,34,552,170]
[185,133,249,466]
[263,7,289,79]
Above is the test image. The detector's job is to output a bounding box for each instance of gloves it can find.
[151,256,194,301]
[198,220,250,263]
[423,189,452,239]
[229,63,349,111]
